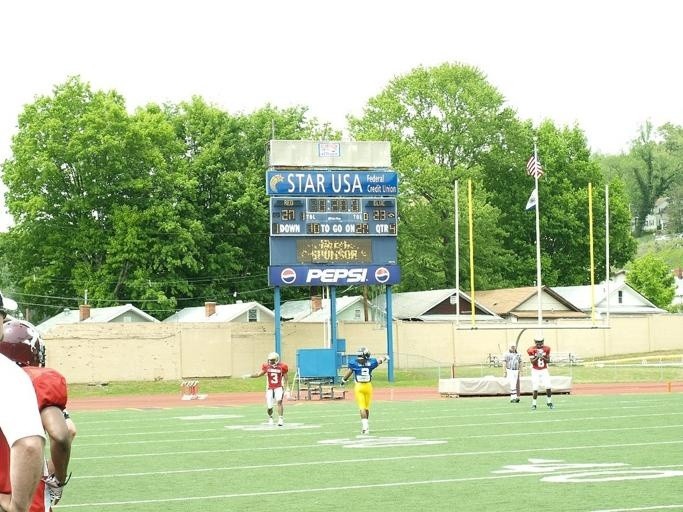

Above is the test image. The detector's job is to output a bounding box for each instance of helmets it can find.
[267,352,280,367]
[534,335,544,348]
[0,293,45,368]
[357,347,371,363]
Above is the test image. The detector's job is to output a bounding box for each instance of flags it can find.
[525,144,543,180]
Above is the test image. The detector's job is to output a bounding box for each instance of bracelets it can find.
[341,377,346,383]
[380,357,386,363]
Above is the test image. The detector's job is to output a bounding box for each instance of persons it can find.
[0,319,74,512]
[527,333,553,410]
[340,346,391,436]
[503,343,525,404]
[243,352,290,427]
[38,340,78,479]
[0,292,47,512]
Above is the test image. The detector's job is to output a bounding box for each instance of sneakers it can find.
[362,423,369,435]
[511,398,519,402]
[532,403,554,410]
[270,418,284,426]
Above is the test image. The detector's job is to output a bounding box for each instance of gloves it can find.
[47,473,63,506]
